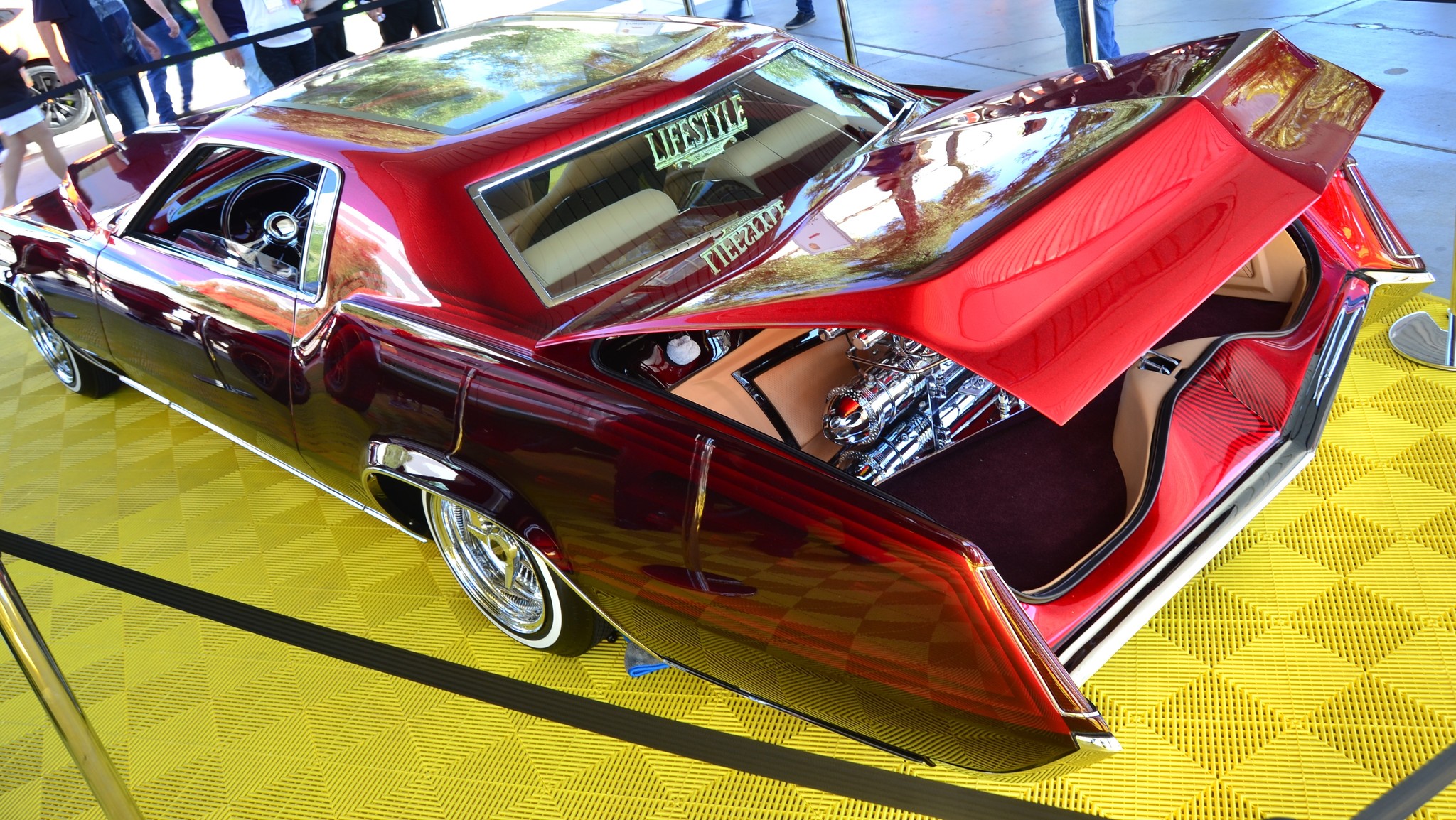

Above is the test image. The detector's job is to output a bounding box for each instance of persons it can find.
[32,0,162,140]
[196,0,443,102]
[0,44,70,212]
[1053,0,1121,68]
[120,0,200,124]
[725,0,818,30]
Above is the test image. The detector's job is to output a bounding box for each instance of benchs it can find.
[512,105,848,300]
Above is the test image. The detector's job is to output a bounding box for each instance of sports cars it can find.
[1,12,1433,753]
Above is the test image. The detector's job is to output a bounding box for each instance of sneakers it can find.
[784,10,816,29]
